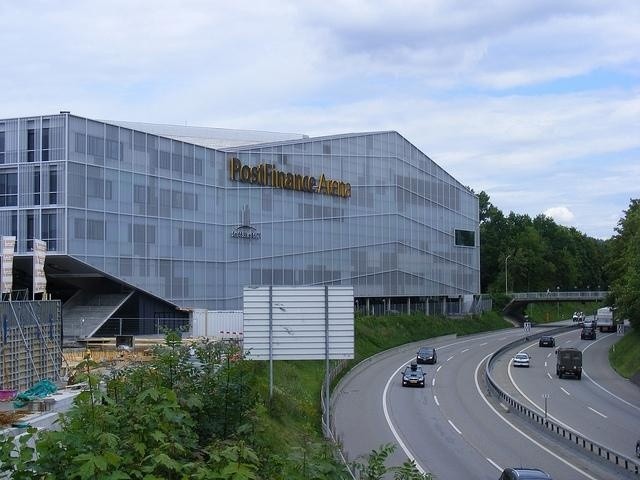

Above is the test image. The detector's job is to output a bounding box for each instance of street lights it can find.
[506,254,511,295]
[355,299,360,319]
[383,299,386,315]
[557,286,560,317]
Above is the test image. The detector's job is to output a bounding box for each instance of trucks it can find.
[555,347,582,380]
[596,307,624,332]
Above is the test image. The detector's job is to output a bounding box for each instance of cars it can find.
[499,467,552,480]
[401,363,427,388]
[572,312,596,340]
[636,440,640,457]
[512,352,531,368]
[416,347,437,365]
[539,336,556,347]
